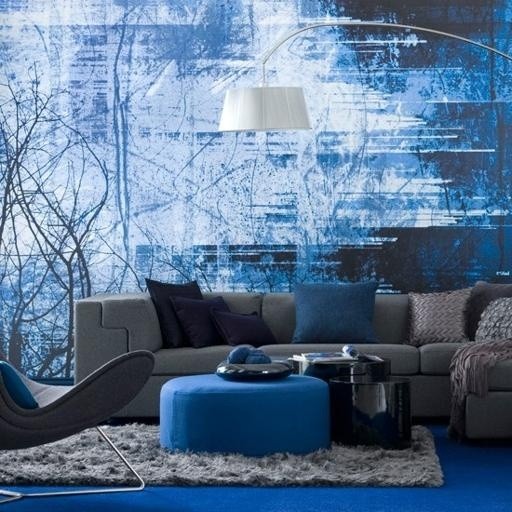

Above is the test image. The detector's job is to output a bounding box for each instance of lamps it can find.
[218,21,511,131]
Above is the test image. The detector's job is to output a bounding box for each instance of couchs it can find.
[73,291,512,439]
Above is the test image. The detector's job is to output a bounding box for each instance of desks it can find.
[159,375,331,456]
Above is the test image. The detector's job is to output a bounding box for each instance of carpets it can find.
[0,423,445,489]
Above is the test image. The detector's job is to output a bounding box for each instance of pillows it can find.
[292,282,379,343]
[1,359,40,407]
[144,278,204,347]
[168,295,230,348]
[211,309,277,345]
[404,287,475,346]
[475,297,511,343]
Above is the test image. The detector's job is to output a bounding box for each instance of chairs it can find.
[0,348,156,504]
